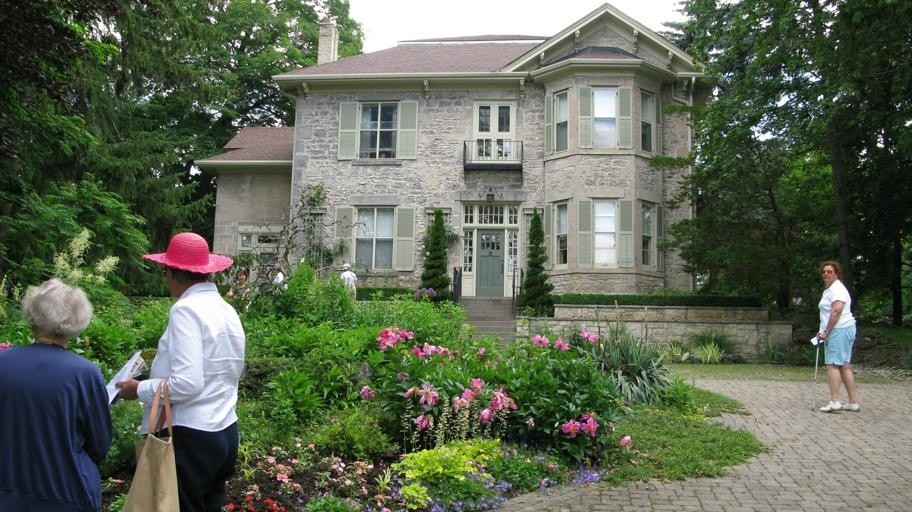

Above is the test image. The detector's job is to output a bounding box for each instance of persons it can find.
[339,263,358,302]
[269,262,288,293]
[814,261,862,414]
[114,231,247,512]
[226,265,253,301]
[1,279,114,512]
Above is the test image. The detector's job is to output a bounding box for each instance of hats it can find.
[142,232,233,274]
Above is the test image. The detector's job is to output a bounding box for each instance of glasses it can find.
[822,270,832,274]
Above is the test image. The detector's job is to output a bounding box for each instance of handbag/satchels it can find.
[121,434,180,511]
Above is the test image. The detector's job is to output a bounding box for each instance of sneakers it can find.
[820,401,843,411]
[842,403,861,411]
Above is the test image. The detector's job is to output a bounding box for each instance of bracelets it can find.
[823,329,829,334]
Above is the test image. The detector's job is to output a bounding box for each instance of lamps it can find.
[486,184,503,205]
